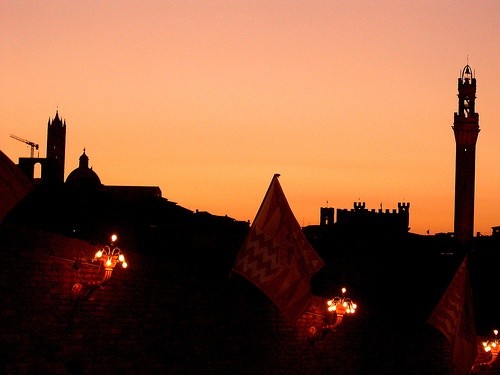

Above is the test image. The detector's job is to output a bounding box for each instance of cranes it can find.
[10,133,39,159]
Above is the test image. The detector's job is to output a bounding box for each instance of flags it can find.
[230,173,325,318]
[424,254,480,375]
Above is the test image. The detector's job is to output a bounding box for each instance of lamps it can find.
[307,286,357,338]
[71,230,130,302]
[468,329,500,375]
